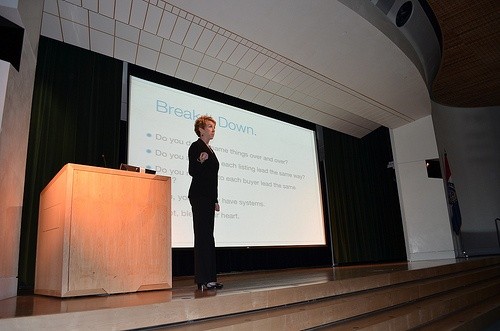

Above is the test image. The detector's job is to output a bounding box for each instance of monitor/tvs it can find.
[120,164,140,172]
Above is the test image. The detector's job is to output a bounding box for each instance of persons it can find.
[187,115,224,290]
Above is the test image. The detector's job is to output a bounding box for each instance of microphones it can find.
[102,155,107,168]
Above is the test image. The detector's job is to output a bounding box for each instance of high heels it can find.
[196,281,223,290]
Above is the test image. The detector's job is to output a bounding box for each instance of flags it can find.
[444,155,462,236]
